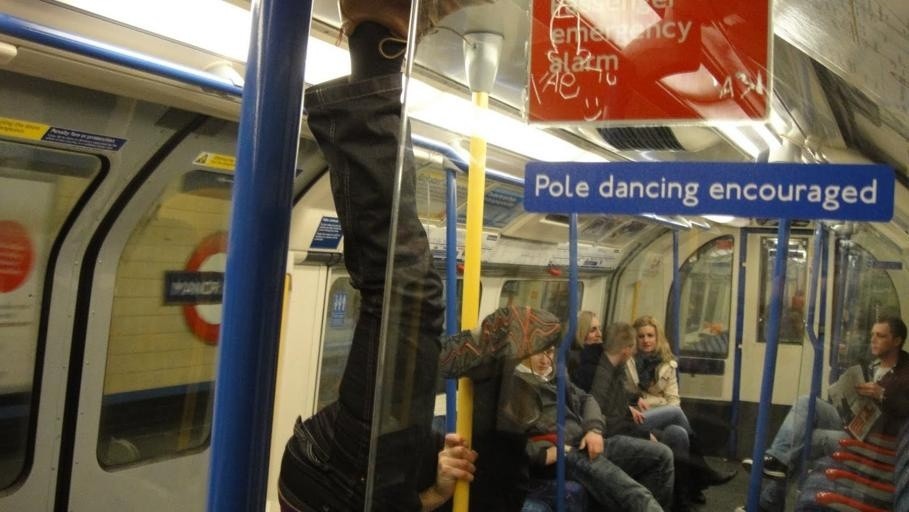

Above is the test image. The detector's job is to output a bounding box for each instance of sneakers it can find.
[735,506,765,512]
[741,455,788,481]
[338,0,495,43]
[439,306,561,380]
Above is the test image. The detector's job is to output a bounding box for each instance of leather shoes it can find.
[689,468,737,503]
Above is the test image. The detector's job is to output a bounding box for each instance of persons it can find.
[740,317,905,512]
[278,2,561,512]
[784,290,806,344]
[511,310,737,512]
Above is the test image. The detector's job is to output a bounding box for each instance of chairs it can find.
[680,329,729,373]
[793,423,908,511]
